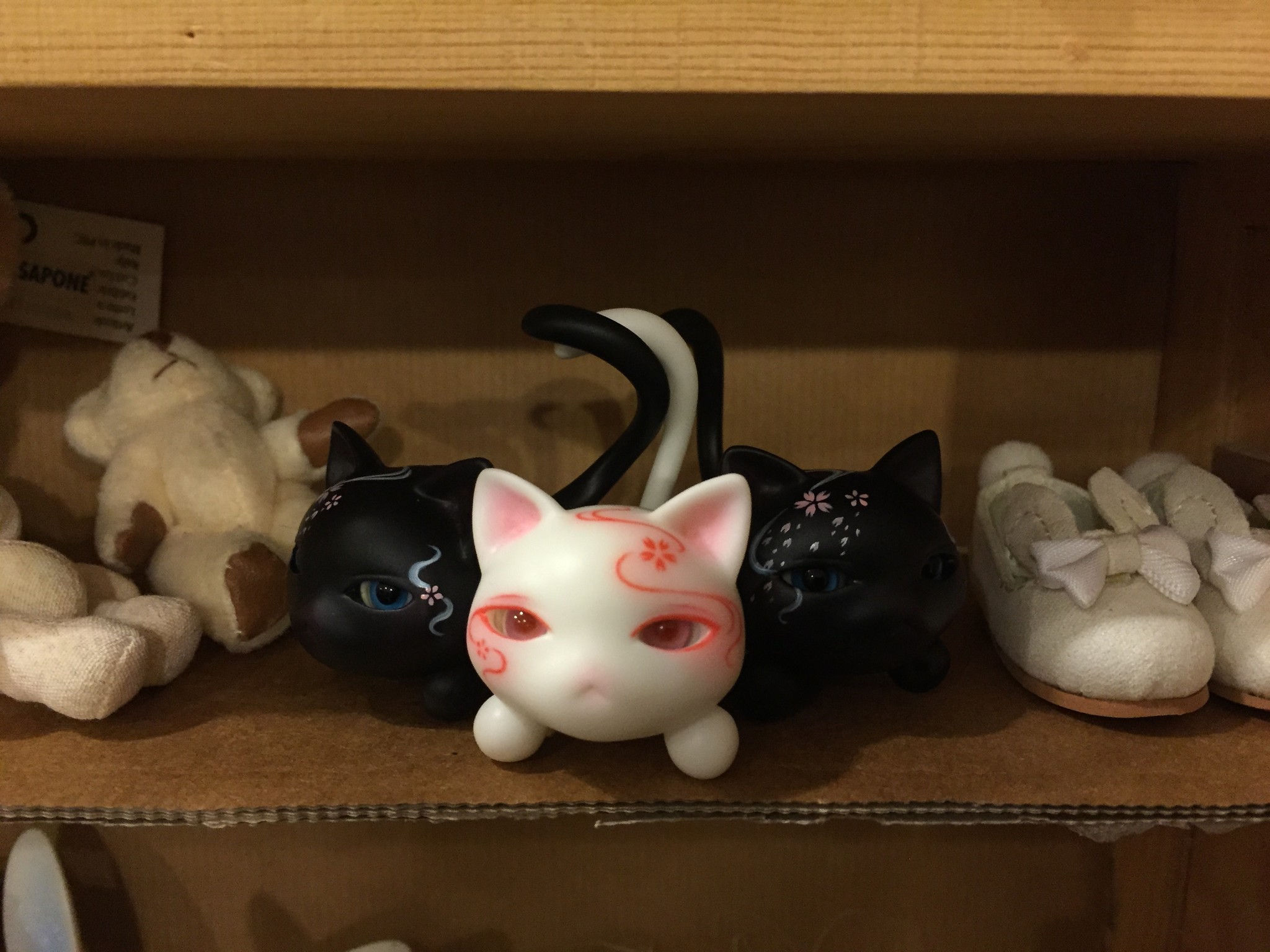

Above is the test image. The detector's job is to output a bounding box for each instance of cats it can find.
[283,303,963,781]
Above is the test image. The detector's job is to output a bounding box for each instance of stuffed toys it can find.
[63,330,379,657]
[0,484,201,720]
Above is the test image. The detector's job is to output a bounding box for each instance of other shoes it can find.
[968,440,1215,728]
[1120,447,1269,728]
